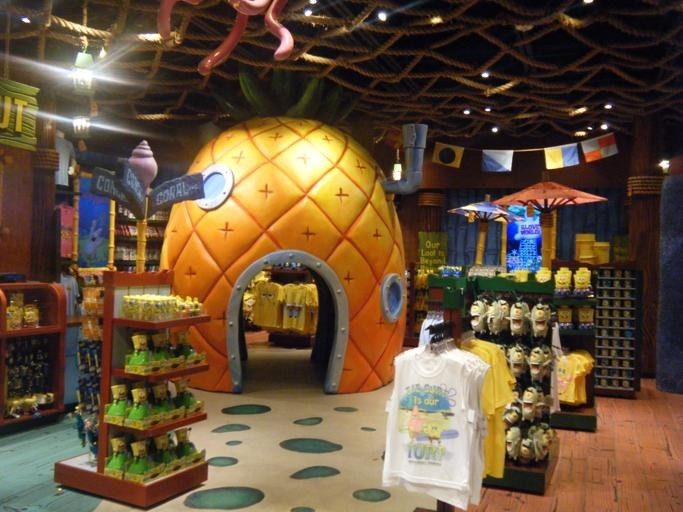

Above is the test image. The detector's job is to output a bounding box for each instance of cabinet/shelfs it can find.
[467,333,561,496]
[54,268,212,504]
[409,258,645,400]
[0,279,68,434]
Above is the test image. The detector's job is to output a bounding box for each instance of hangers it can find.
[392,318,488,373]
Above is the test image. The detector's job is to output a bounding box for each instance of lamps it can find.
[73,46,94,89]
[392,149,402,181]
